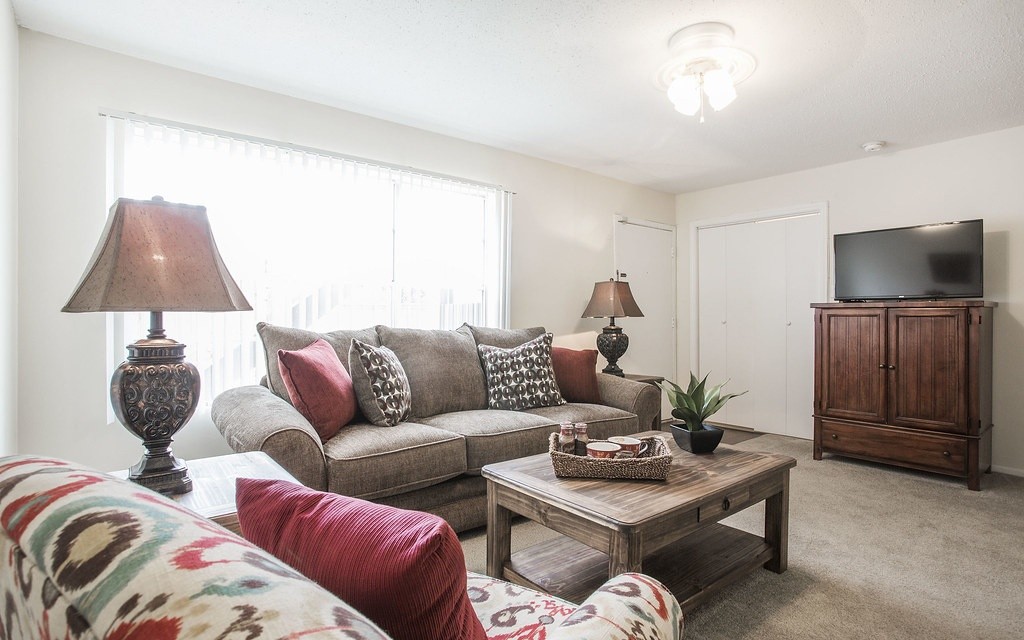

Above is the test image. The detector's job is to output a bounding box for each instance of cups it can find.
[607,436,648,458]
[586,442,621,459]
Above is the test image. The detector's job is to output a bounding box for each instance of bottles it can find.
[559,422,588,456]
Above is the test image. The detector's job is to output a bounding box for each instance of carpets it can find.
[456,433,1024,640]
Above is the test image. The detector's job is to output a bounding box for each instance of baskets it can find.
[549,432,674,482]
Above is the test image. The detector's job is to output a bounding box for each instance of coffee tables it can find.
[462,428,800,614]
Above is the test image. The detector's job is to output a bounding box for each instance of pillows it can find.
[549,344,600,405]
[274,337,355,443]
[343,338,415,429]
[474,333,567,409]
[232,471,493,640]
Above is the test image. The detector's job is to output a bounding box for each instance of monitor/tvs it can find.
[834,218,984,304]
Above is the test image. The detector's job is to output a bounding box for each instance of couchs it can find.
[211,317,667,535]
[0,449,686,640]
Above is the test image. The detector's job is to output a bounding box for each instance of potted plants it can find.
[648,371,752,454]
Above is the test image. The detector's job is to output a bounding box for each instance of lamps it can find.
[581,276,646,384]
[59,194,257,501]
[661,23,752,124]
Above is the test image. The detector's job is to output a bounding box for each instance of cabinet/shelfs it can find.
[617,367,666,430]
[803,299,999,493]
[103,449,307,537]
